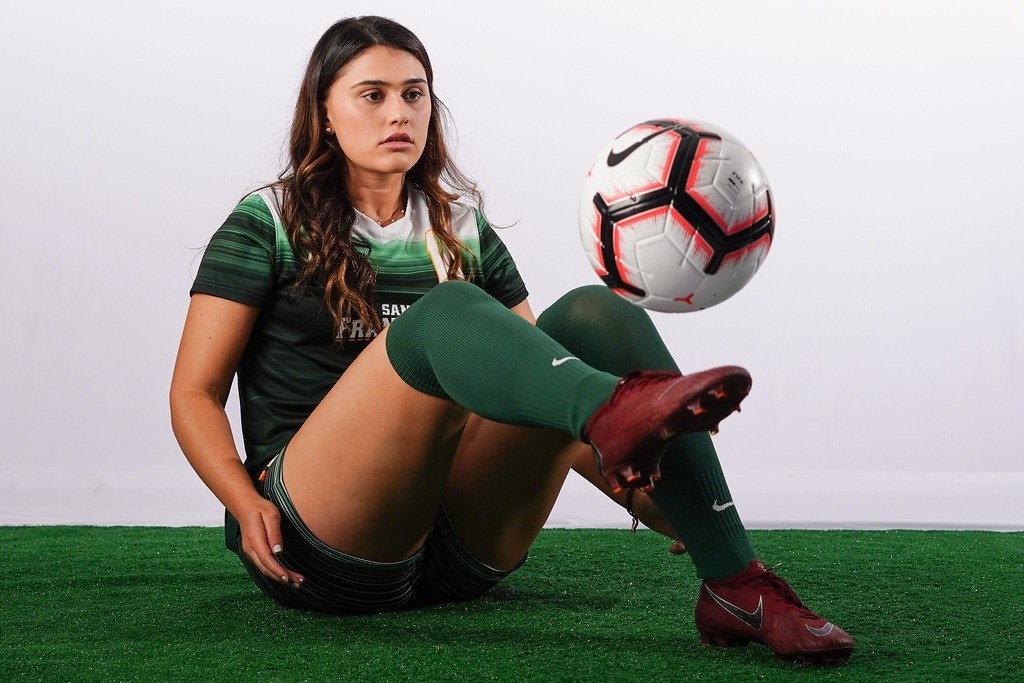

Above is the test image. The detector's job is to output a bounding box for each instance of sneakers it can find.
[582,365,753,497]
[695,560,856,666]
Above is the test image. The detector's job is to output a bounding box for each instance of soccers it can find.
[577,114,775,314]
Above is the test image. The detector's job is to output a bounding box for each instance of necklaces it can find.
[355,202,405,226]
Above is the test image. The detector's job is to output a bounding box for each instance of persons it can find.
[168,16,856,663]
